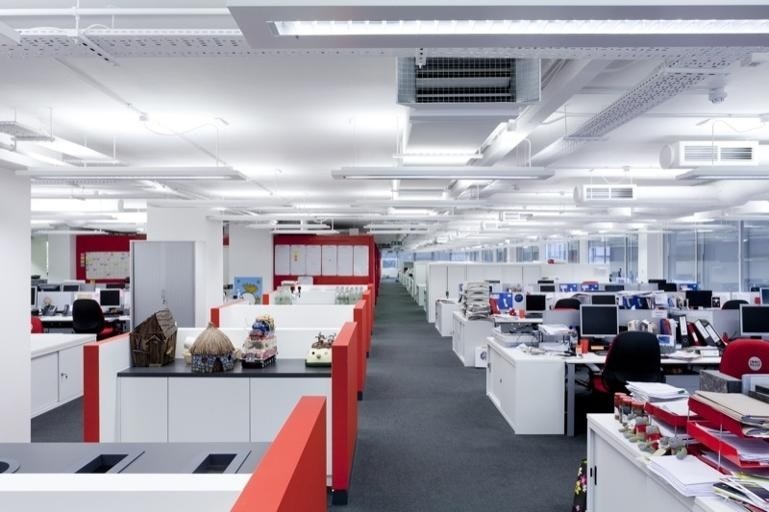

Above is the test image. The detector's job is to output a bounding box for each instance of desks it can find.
[435,299,457,337]
[452,312,543,367]
[31,309,130,336]
[485,336,721,435]
[397,271,427,312]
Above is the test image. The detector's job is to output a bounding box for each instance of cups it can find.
[580,338,589,354]
[519,309,525,318]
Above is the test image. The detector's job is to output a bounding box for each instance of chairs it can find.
[72,299,126,340]
[555,299,581,309]
[31,315,44,333]
[574,331,666,412]
[721,299,749,309]
[719,338,769,379]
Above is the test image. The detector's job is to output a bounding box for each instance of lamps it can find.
[148,197,338,234]
[15,166,247,182]
[354,199,486,235]
[226,0,769,52]
[675,167,769,181]
[330,166,556,180]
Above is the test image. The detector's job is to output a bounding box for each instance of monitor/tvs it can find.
[39,283,61,291]
[591,294,616,305]
[539,283,556,292]
[61,285,81,291]
[579,304,620,339]
[760,287,769,304]
[537,279,555,284]
[605,284,625,292]
[686,291,712,310]
[99,288,121,314]
[739,303,769,338]
[525,294,547,313]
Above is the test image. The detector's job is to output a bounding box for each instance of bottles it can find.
[570,321,578,348]
[296,286,303,304]
[614,392,646,426]
[334,286,363,305]
[754,296,760,305]
[289,287,296,304]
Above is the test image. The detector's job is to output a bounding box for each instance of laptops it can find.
[31,286,38,311]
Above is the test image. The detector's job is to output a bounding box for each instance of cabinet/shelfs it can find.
[585,413,745,512]
[30,332,97,420]
[0,441,273,473]
[115,352,332,489]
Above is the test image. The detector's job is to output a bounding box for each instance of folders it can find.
[694,320,716,345]
[668,314,689,347]
[702,320,726,348]
[687,322,700,345]
